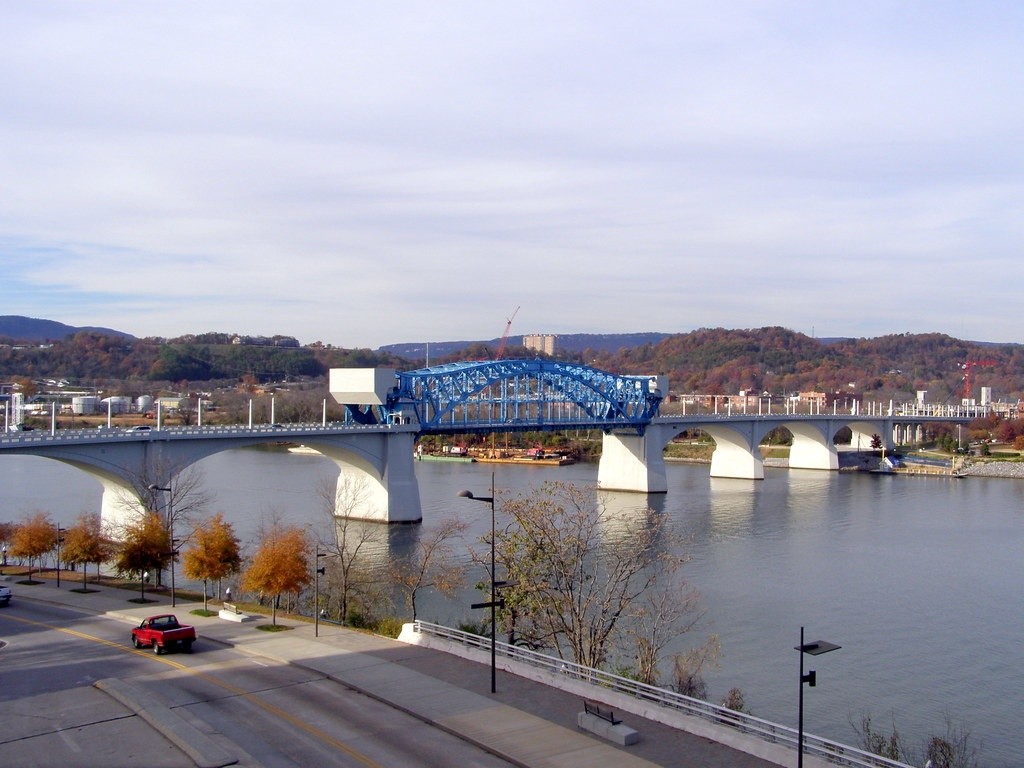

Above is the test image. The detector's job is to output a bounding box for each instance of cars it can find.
[126,426,152,433]
[0,585,13,604]
[268,424,285,429]
[953,471,967,478]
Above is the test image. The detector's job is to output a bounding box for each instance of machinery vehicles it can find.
[496,306,520,359]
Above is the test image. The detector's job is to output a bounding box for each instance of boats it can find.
[471,432,576,465]
[957,359,999,398]
[417,443,474,462]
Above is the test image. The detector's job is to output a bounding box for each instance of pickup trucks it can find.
[131,614,196,654]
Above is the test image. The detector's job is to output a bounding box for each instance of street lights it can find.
[148,484,175,607]
[456,489,496,693]
